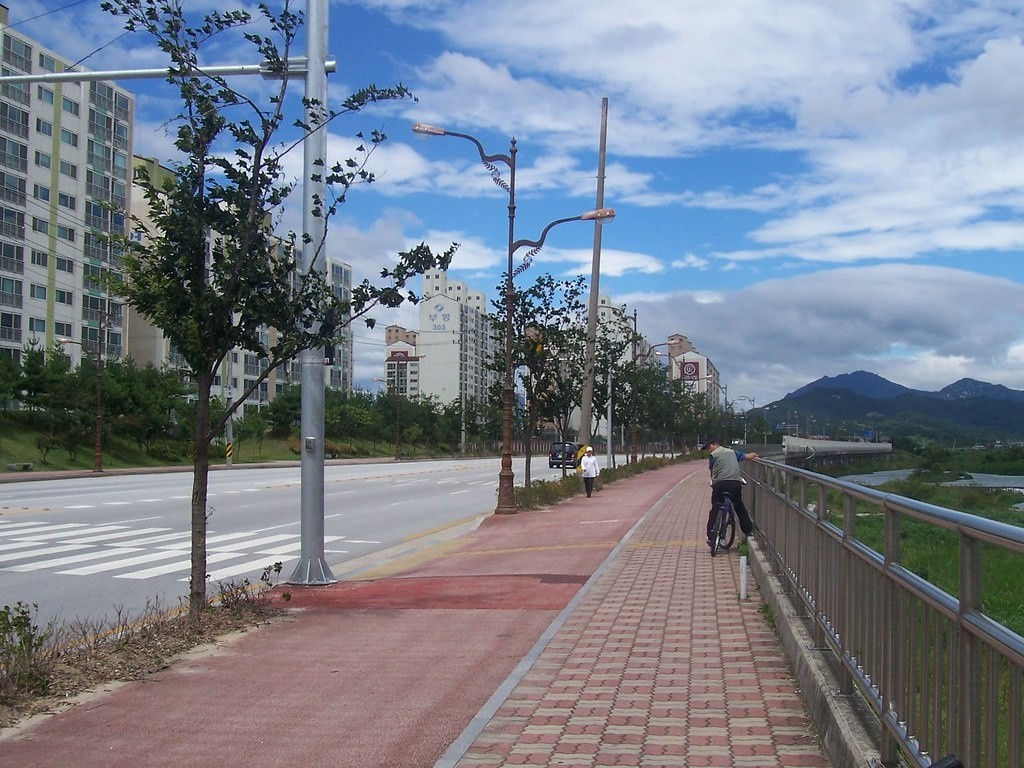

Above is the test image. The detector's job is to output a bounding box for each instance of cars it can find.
[693,444,706,452]
[732,438,743,445]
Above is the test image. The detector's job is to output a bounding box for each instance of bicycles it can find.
[707,476,749,558]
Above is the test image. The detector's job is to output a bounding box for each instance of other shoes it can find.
[586,493,590,497]
[706,538,714,547]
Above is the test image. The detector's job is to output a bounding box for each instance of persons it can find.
[581,446,601,498]
[700,437,757,547]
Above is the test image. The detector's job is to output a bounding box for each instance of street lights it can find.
[411,120,615,515]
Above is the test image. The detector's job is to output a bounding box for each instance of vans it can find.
[549,441,579,469]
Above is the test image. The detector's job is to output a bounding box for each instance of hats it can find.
[699,437,716,450]
[587,447,592,452]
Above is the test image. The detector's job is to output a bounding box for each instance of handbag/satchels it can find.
[595,477,603,492]
